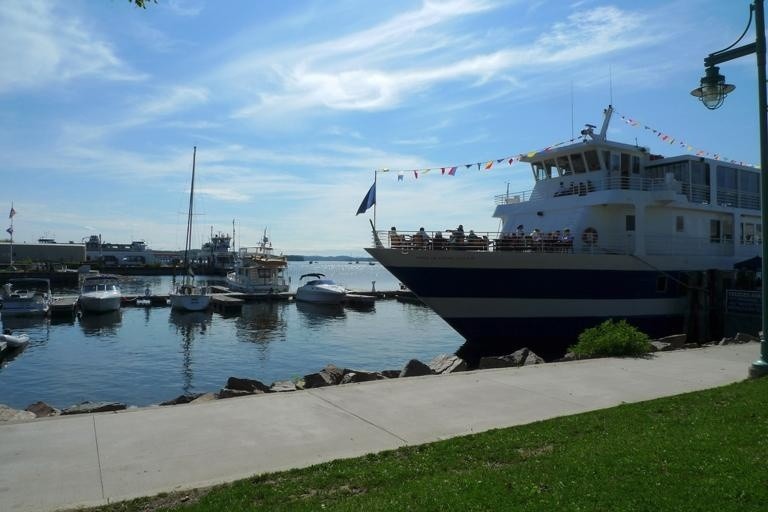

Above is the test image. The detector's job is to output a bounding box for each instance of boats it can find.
[77,309,122,337]
[225,226,289,294]
[297,300,345,327]
[207,218,238,277]
[0,342,29,370]
[293,274,349,307]
[2,278,53,316]
[236,299,286,361]
[0,334,31,355]
[1,310,50,349]
[308,259,376,266]
[362,105,764,360]
[80,274,122,314]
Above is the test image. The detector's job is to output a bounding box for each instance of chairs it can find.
[388,231,578,257]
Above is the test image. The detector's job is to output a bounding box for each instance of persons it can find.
[553,180,595,197]
[391,225,594,253]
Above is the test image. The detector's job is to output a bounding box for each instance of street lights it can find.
[690,0,768,378]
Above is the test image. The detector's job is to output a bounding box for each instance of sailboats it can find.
[168,146,212,311]
[168,308,212,397]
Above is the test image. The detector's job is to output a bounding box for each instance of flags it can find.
[356,181,375,216]
